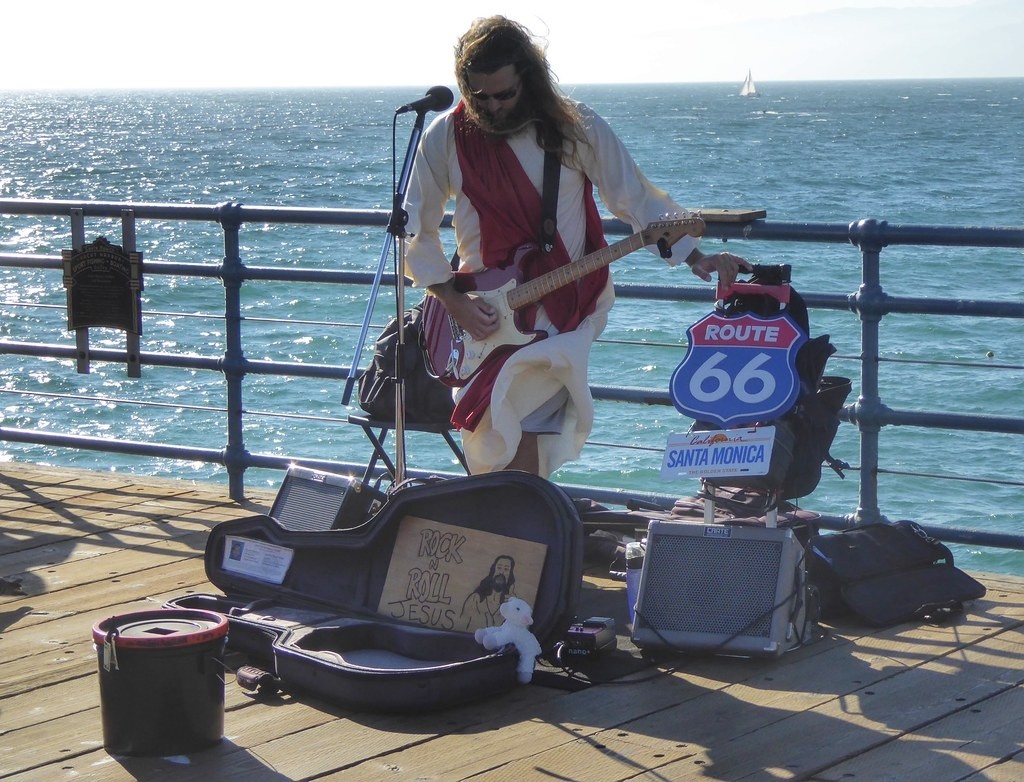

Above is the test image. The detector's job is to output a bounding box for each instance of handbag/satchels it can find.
[805,519,986,629]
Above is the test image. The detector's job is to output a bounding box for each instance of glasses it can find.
[469,75,522,101]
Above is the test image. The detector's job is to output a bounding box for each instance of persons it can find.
[229,543,242,561]
[397,13,754,480]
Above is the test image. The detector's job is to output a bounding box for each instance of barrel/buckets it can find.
[90,608,229,758]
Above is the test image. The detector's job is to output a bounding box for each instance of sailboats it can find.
[738,69,760,98]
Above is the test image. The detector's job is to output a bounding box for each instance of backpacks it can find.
[357,298,457,423]
[716,264,852,499]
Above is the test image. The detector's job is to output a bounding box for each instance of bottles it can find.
[625,541,646,624]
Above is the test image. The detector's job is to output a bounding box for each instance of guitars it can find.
[422,208,708,388]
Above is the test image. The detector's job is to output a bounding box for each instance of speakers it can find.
[267,462,390,532]
[629,517,808,656]
[395,84,455,114]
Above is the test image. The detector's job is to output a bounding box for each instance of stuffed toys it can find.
[475,598,542,684]
